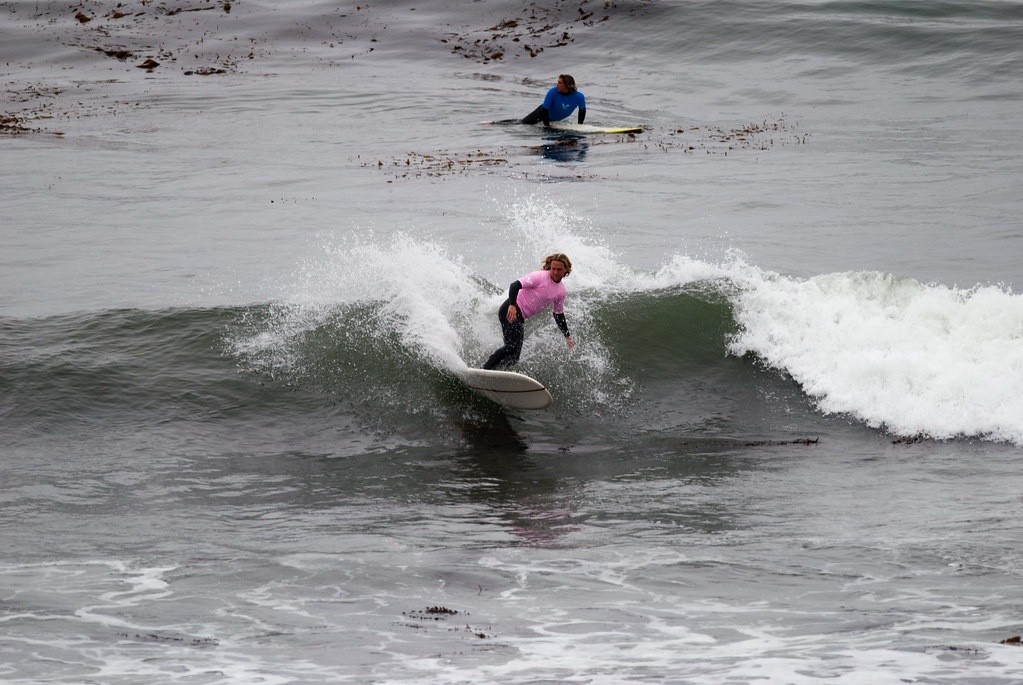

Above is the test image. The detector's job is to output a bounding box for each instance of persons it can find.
[484,254,575,369]
[524,75,586,125]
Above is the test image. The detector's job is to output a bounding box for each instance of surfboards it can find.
[444,354,555,411]
[480,116,643,135]
